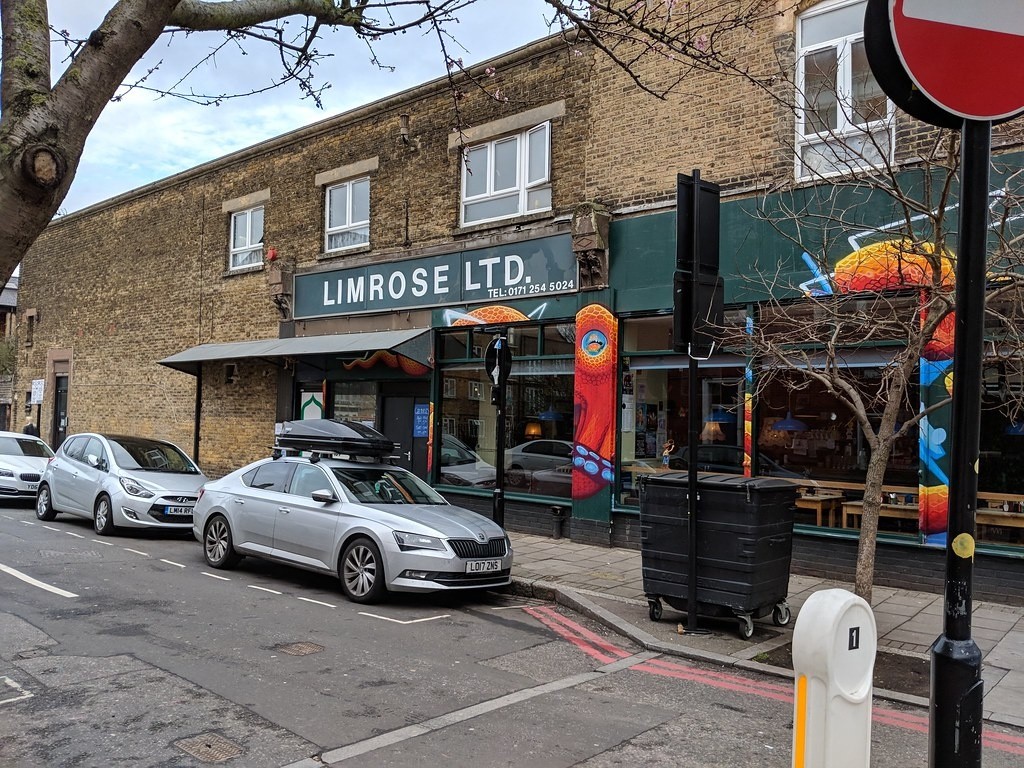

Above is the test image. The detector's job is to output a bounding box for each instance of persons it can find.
[23,417,39,435]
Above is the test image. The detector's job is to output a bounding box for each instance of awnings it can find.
[159,327,432,377]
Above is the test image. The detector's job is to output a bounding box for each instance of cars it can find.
[504,441,576,487]
[192,456,514,605]
[441,433,493,489]
[670,442,803,479]
[36,432,211,536]
[0,431,56,499]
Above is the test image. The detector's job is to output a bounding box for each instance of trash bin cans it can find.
[636,470,800,640]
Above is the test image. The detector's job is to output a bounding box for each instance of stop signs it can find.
[887,0,1024,118]
